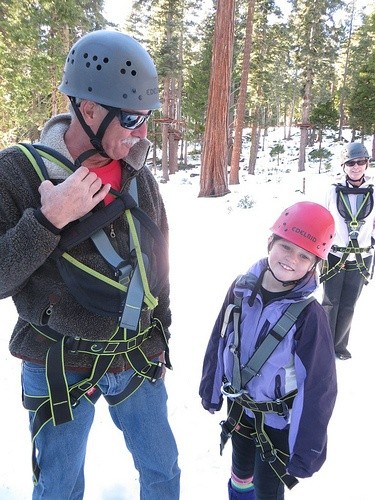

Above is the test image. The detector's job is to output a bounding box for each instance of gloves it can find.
[202,394,224,411]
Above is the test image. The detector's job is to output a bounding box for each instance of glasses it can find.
[98,103,151,130]
[345,160,366,166]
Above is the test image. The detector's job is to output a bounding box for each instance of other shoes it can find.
[337,348,351,359]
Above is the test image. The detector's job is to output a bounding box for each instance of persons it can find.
[198,201,338,500]
[317,142,375,360]
[0,30,180,500]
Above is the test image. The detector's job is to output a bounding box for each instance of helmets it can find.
[269,202,335,261]
[341,143,369,166]
[58,30,161,109]
